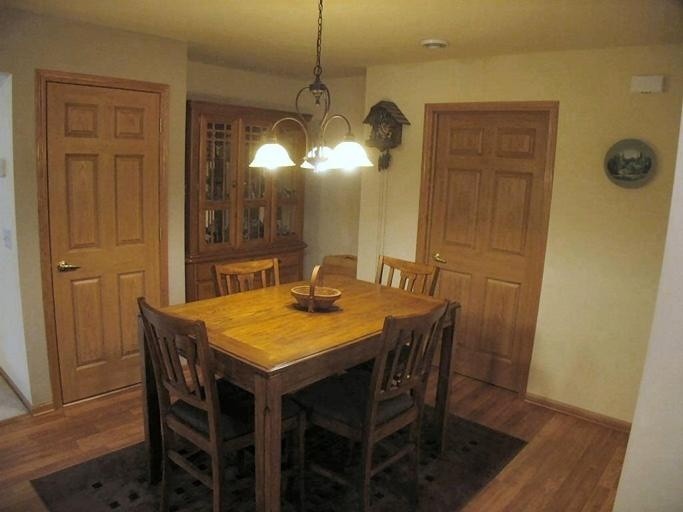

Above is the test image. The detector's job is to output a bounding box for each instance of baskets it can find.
[290,265,342,312]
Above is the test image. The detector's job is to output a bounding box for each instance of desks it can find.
[140,274,461,511]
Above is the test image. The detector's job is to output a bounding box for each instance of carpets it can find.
[30,403,527,512]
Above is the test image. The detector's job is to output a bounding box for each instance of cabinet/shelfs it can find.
[185,99,313,300]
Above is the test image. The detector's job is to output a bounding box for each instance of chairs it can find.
[210,257,281,294]
[298,300,449,512]
[346,256,442,397]
[137,296,305,511]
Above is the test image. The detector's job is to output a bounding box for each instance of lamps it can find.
[249,0,374,175]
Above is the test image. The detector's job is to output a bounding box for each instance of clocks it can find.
[362,100,411,172]
